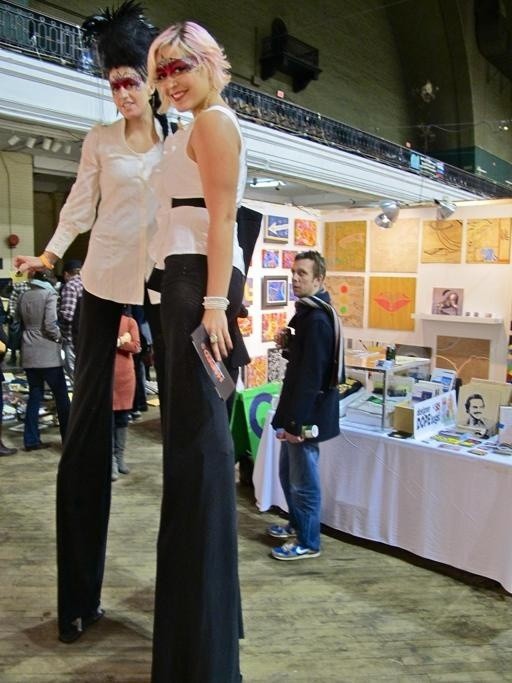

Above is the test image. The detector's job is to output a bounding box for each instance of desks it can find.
[251,390,511,596]
[344,353,433,430]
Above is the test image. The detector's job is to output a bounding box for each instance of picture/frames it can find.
[262,273,290,307]
[263,212,290,242]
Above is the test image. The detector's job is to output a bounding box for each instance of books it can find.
[337,347,512,451]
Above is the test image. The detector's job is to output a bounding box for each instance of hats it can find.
[62,258,82,271]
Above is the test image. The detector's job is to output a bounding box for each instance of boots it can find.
[115,425,130,474]
[112,427,120,482]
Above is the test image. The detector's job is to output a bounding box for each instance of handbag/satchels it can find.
[9,290,29,350]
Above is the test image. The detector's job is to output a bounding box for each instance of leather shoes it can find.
[25,443,51,450]
[0,441,17,456]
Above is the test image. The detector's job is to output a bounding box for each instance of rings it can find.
[210,333,217,342]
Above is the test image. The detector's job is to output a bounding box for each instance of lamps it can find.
[372,199,403,228]
[432,196,460,217]
[8,123,73,154]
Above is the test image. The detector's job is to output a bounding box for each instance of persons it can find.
[264,250,341,560]
[147,19,261,683]
[0,259,162,479]
[13,44,245,643]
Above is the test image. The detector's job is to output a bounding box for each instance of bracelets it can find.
[203,296,230,311]
[40,254,56,270]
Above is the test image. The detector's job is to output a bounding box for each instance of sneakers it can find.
[268,524,297,540]
[132,411,142,421]
[271,542,321,562]
